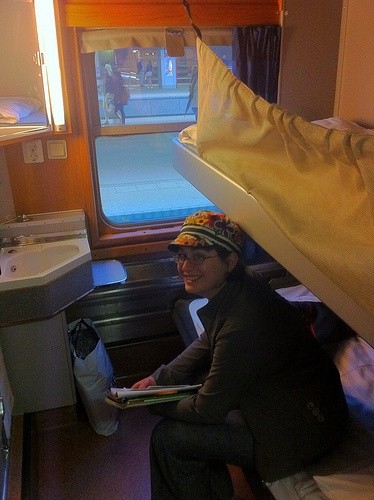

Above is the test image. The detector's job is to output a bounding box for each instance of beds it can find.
[171,277,374,500]
[172,118,374,349]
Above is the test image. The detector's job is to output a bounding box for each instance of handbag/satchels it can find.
[67,318,118,436]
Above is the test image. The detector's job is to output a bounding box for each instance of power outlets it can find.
[21,139,44,163]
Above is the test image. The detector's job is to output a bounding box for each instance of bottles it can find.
[168,60,172,75]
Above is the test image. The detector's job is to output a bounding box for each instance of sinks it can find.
[0,237,95,325]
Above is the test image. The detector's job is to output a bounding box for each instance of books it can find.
[104,384,200,410]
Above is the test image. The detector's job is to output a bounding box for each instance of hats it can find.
[167,209,244,257]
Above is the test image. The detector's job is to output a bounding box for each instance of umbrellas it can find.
[184,89,193,117]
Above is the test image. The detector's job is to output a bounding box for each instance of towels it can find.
[1,97,42,125]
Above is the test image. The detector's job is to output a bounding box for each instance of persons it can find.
[131,210,349,500]
[138,59,145,87]
[103,63,128,125]
[146,60,154,89]
[189,64,198,122]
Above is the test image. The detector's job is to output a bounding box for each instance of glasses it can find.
[173,252,223,264]
